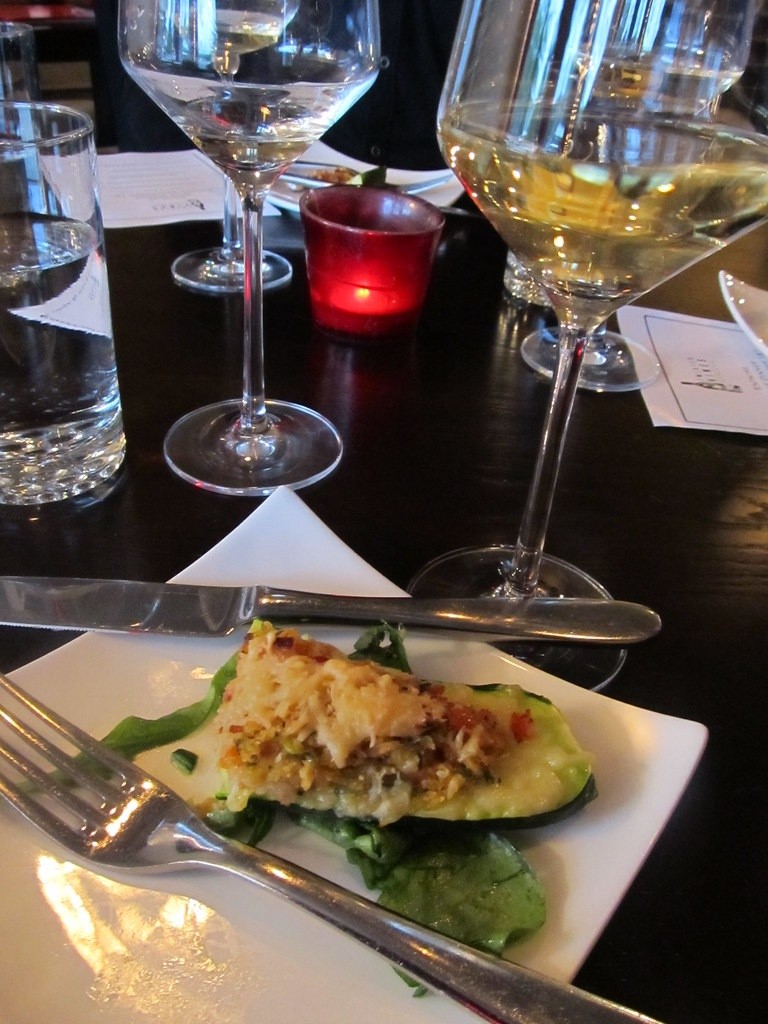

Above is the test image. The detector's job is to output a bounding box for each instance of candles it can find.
[299,182,447,344]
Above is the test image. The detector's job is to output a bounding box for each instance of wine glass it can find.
[113,3,389,498]
[170,156,294,293]
[520,0,760,396]
[408,0,765,695]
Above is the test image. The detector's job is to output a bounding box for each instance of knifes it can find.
[1,574,667,645]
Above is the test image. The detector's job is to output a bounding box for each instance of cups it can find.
[500,246,556,309]
[0,19,43,108]
[1,103,132,507]
[298,185,447,346]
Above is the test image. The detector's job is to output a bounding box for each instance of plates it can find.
[265,136,467,220]
[1,486,710,1023]
[718,267,768,361]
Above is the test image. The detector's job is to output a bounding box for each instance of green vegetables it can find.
[5,616,548,994]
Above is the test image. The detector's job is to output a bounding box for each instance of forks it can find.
[0,676,661,1024]
